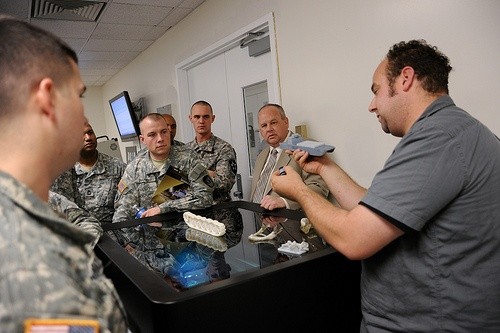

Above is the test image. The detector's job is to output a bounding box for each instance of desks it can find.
[98,201,363,333]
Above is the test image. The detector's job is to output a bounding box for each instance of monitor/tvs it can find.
[108,90,141,142]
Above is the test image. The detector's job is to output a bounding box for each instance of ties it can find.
[252,149,278,203]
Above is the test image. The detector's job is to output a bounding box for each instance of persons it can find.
[271,39,500,333]
[0,16,133,333]
[104,210,286,293]
[48,102,329,223]
